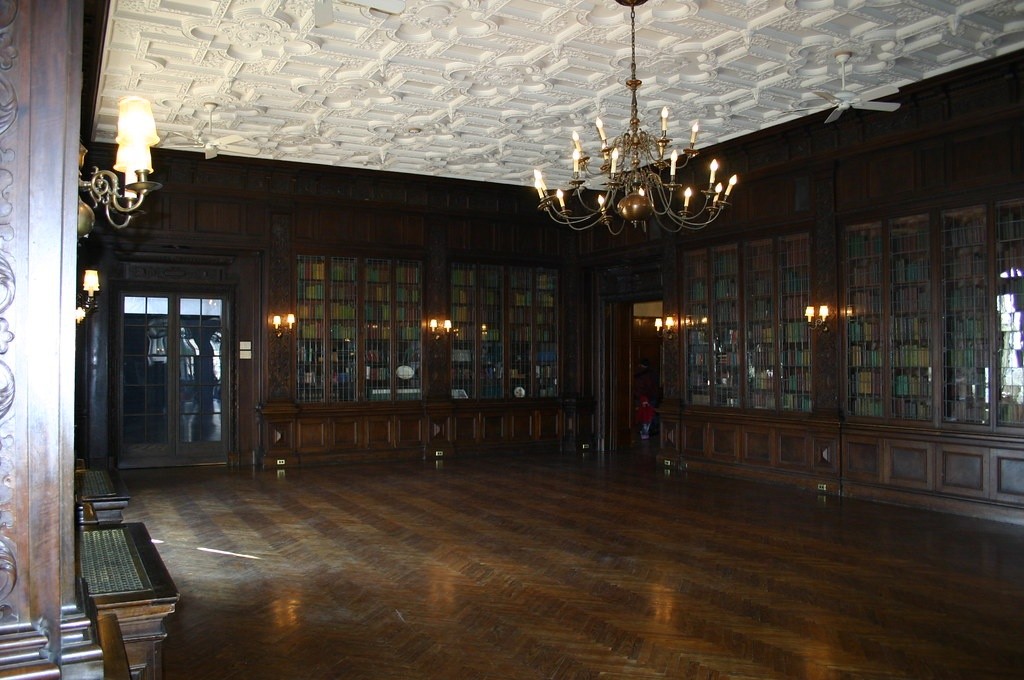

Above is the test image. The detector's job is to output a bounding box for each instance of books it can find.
[297,255,557,401]
[689,214,1024,425]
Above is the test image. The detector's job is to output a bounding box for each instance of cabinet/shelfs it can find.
[295,254,559,400]
[685,197,1024,424]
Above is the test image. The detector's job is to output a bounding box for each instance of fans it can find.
[172,102,245,159]
[783,52,901,124]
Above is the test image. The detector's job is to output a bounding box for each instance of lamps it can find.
[429,319,453,338]
[272,313,295,337]
[654,316,674,340]
[804,304,829,332]
[76,269,101,324]
[79,95,162,237]
[533,0,738,234]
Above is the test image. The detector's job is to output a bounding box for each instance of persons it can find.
[636,395,654,439]
